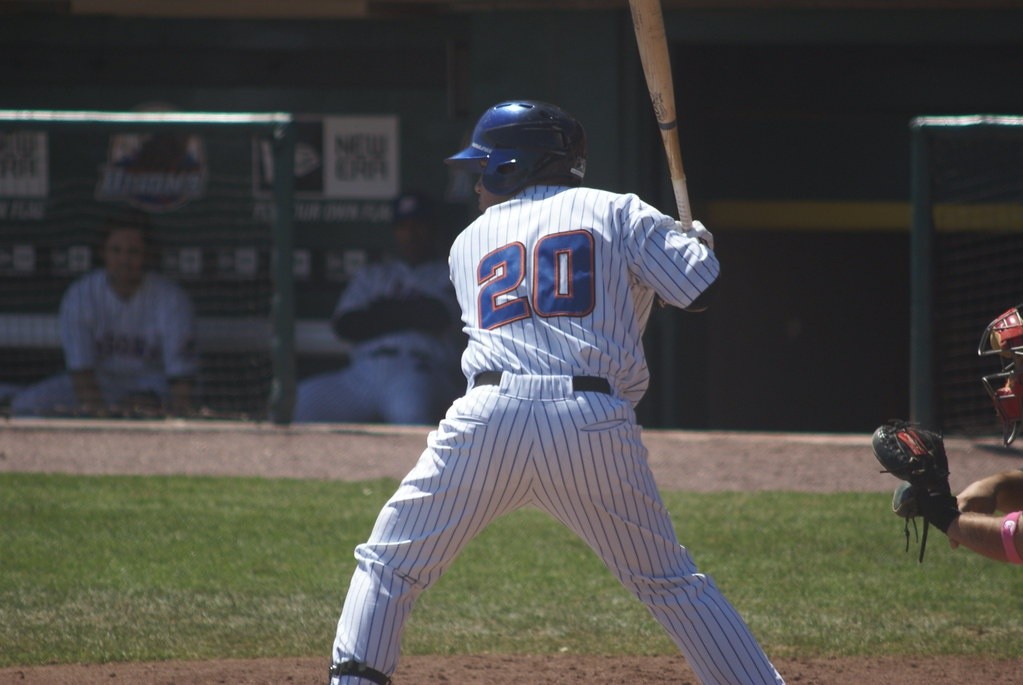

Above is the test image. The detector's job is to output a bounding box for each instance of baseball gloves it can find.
[870,418,963,520]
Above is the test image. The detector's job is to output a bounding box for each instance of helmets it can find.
[444,101,587,195]
[977,304,1023,448]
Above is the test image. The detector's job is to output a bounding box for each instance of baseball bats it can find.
[627,0,693,234]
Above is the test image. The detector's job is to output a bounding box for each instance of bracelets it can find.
[1000,511,1023,563]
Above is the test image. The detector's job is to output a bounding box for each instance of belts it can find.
[472,372,610,396]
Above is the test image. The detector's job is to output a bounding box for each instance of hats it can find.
[396,192,429,218]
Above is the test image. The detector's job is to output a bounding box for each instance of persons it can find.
[870,302,1023,563]
[328,98,785,685]
[245,190,459,424]
[8,202,205,416]
